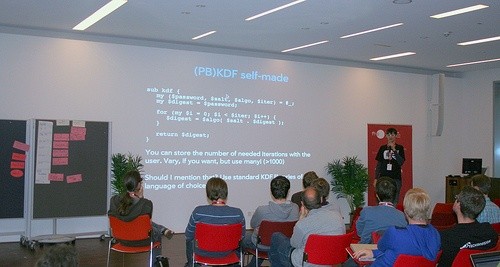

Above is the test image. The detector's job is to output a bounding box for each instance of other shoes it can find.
[162,228,174,240]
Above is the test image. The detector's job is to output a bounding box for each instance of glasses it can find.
[137,178,143,185]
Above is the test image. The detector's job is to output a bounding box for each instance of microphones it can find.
[389,146,391,154]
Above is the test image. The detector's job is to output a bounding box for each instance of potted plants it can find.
[325,155,370,228]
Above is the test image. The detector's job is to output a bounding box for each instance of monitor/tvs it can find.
[462,157,483,177]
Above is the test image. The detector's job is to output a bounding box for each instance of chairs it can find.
[243,220,297,267]
[302,232,354,267]
[192,222,244,267]
[346,200,500,267]
[106,213,160,267]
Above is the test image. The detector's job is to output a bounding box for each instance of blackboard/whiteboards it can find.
[0,119,27,219]
[34,119,110,218]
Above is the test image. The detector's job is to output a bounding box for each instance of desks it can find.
[346,247,374,267]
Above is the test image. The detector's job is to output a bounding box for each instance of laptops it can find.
[470,251,500,267]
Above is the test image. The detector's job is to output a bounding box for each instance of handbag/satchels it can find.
[153,256,169,267]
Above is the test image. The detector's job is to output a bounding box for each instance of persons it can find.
[437,186,499,267]
[309,178,341,213]
[269,186,346,267]
[373,128,405,208]
[108,171,174,267]
[356,175,408,244]
[470,174,500,225]
[248,176,300,267]
[184,177,246,267]
[291,171,319,208]
[345,188,442,267]
